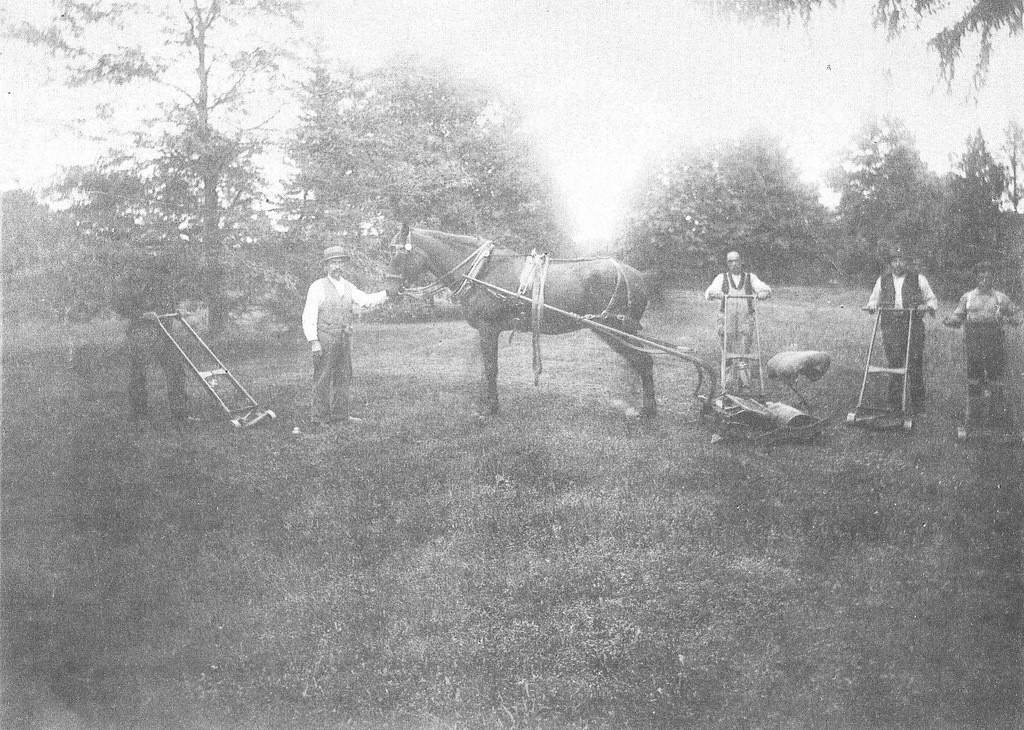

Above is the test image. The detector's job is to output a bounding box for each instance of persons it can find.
[946,265,1020,418]
[867,243,938,417]
[302,246,400,431]
[704,251,772,389]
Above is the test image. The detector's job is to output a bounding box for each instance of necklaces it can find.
[728,271,745,290]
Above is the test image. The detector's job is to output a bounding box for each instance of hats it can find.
[321,246,350,263]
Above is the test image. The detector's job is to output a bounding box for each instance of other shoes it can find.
[341,415,362,422]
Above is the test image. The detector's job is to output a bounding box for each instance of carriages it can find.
[384,223,832,446]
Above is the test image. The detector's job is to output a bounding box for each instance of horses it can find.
[382,221,660,419]
